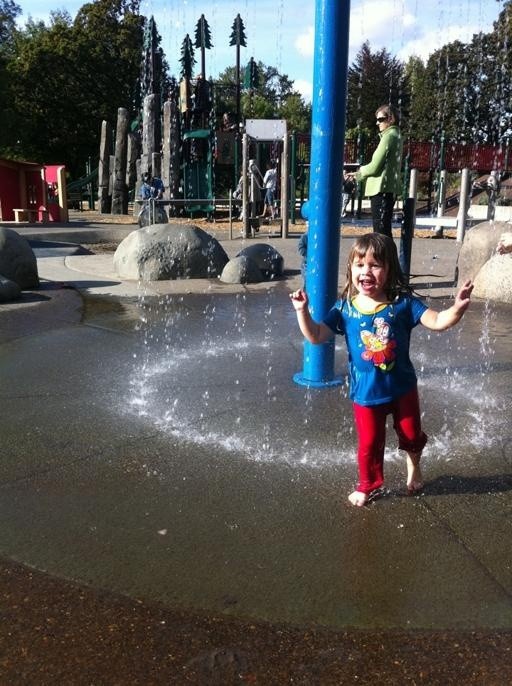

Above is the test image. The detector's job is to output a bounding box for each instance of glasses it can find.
[377,117,387,122]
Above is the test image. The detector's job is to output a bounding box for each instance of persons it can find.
[138,171,165,214]
[298,220,310,292]
[231,161,279,222]
[345,103,404,239]
[341,170,354,218]
[287,231,474,507]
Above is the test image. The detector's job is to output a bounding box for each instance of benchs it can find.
[13,208,49,223]
